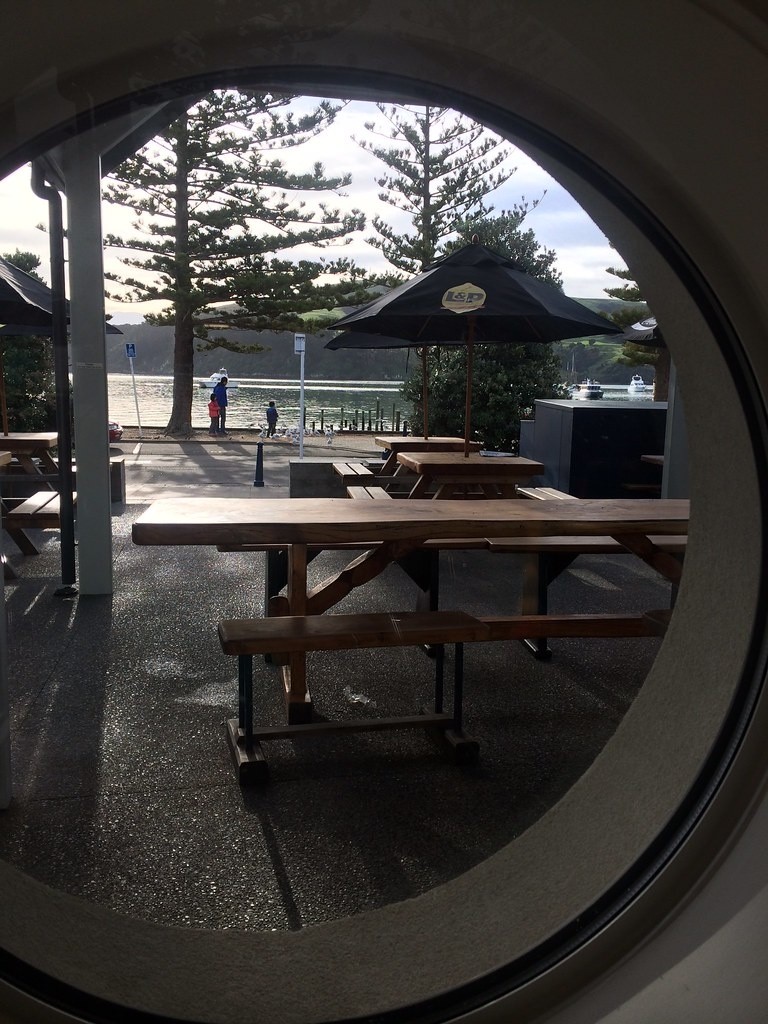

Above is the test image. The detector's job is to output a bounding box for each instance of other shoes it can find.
[208,433,217,437]
[221,431,229,435]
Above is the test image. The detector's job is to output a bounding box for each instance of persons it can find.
[208,377,228,436]
[266,401,278,438]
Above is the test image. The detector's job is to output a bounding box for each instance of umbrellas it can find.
[322,233,627,460]
[1,256,123,436]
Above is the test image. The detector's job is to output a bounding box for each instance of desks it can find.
[0,451,61,555]
[0,432,60,492]
[131,499,691,714]
[379,451,545,500]
[339,436,482,491]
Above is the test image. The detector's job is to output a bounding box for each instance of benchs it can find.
[488,533,689,659]
[216,539,491,662]
[345,484,394,500]
[468,609,675,641]
[332,460,376,479]
[516,486,581,501]
[9,491,78,519]
[218,611,485,786]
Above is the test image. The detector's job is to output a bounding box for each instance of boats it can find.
[193,368,240,388]
[567,377,604,398]
[628,374,646,392]
